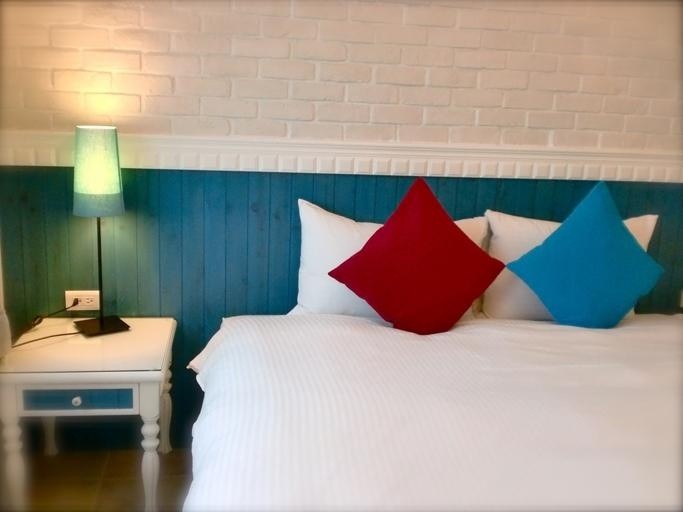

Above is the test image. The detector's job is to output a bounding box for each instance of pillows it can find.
[328,179,505,334]
[486,203,659,324]
[287,195,489,317]
[509,181,668,328]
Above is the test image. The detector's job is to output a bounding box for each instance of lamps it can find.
[73,127,130,336]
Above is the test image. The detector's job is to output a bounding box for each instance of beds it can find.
[182,315,683,510]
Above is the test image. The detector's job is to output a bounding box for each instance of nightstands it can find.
[5,316,177,512]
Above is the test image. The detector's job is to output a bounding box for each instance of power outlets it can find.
[67,292,99,310]
